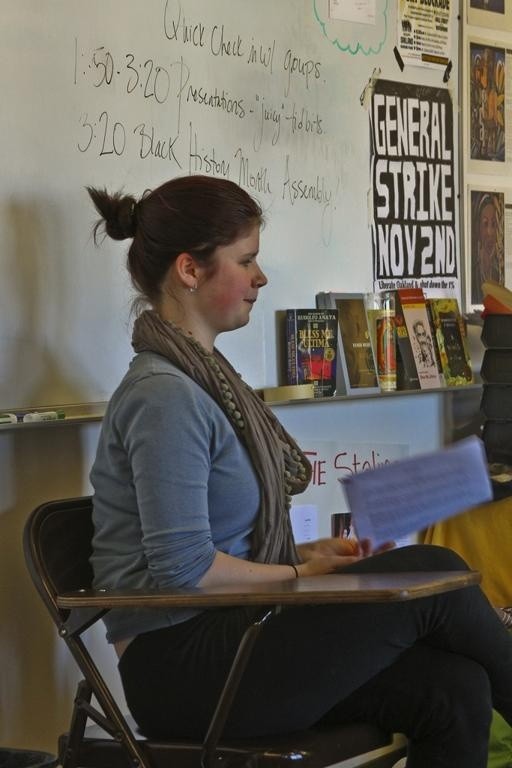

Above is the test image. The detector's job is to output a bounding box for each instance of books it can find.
[283,286,474,397]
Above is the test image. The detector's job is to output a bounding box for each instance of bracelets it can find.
[289,562,299,578]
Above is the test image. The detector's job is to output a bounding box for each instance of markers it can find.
[0,408,67,424]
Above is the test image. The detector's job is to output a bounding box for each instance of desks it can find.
[56,570,479,767]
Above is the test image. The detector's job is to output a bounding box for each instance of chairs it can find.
[26,496,483,766]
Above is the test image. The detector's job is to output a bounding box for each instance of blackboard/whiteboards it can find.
[0,0,459,430]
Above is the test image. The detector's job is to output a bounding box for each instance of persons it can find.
[82,175,512,768]
[474,194,503,299]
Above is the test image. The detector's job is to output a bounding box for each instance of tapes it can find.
[260,384,314,401]
